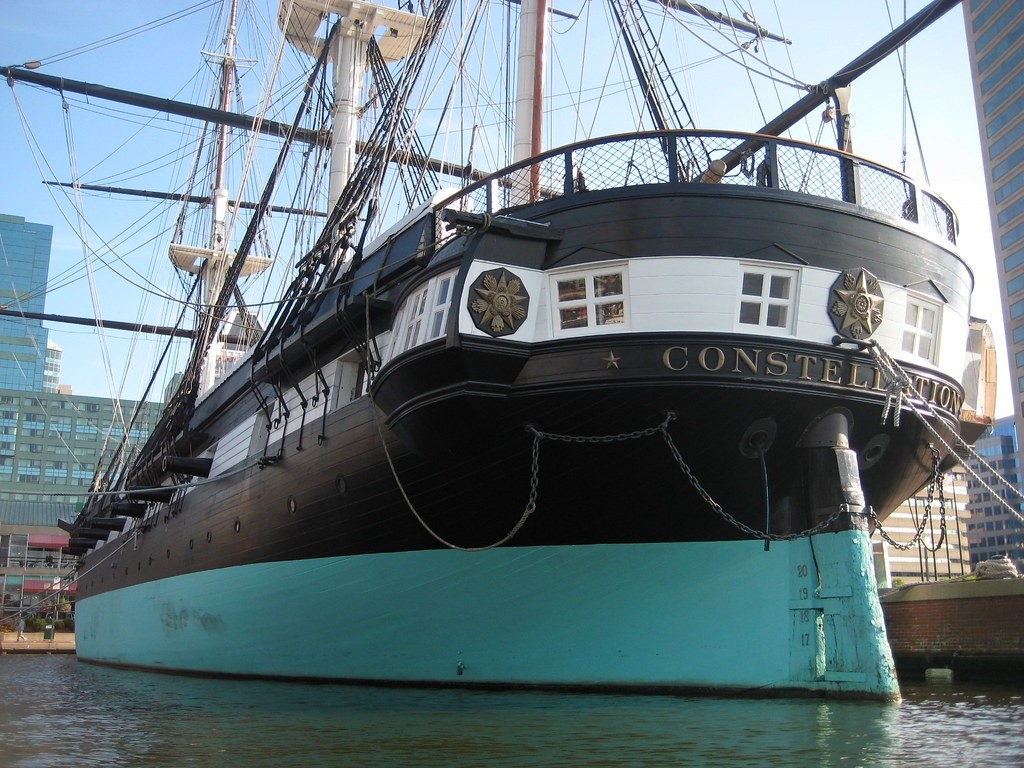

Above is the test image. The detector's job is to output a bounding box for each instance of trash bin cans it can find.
[44,623,55,639]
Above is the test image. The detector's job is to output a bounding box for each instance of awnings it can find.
[23,580,77,594]
[28,533,71,548]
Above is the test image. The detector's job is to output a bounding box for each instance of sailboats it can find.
[10,1,964,704]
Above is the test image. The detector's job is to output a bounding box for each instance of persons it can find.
[15,617,27,642]
